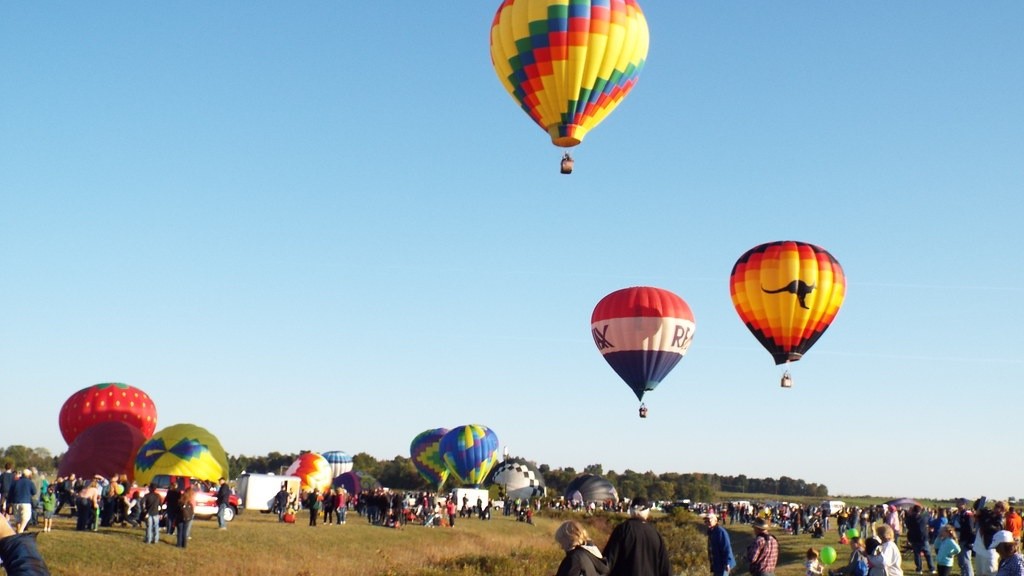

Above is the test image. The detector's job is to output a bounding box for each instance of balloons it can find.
[847,528,858,539]
[819,545,836,564]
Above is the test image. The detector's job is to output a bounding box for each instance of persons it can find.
[163,477,229,548]
[721,502,804,535]
[547,497,624,514]
[274,485,295,523]
[803,505,833,538]
[627,499,721,523]
[745,517,780,576]
[354,487,410,528]
[307,487,352,526]
[0,462,81,576]
[457,494,493,520]
[503,492,538,526]
[703,513,737,576]
[829,498,1024,576]
[413,490,460,527]
[805,547,824,576]
[601,497,673,576]
[76,473,163,544]
[554,520,610,576]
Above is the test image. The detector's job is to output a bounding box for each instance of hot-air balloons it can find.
[285,451,354,496]
[490,458,548,499]
[56,383,229,490]
[439,424,499,488]
[489,0,650,175]
[411,428,451,492]
[591,286,697,418]
[565,473,619,510]
[731,241,846,388]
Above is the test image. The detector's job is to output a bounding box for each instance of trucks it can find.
[822,500,846,517]
[238,474,301,514]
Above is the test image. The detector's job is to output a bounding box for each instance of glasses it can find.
[704,519,714,524]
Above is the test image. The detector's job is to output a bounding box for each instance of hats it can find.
[988,529,1013,549]
[23,468,32,477]
[631,496,653,512]
[752,517,771,529]
[704,514,717,521]
[888,505,897,512]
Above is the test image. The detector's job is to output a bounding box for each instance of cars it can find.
[128,474,244,521]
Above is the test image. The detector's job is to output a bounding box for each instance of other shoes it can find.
[829,569,836,576]
[916,570,922,575]
[930,570,938,574]
